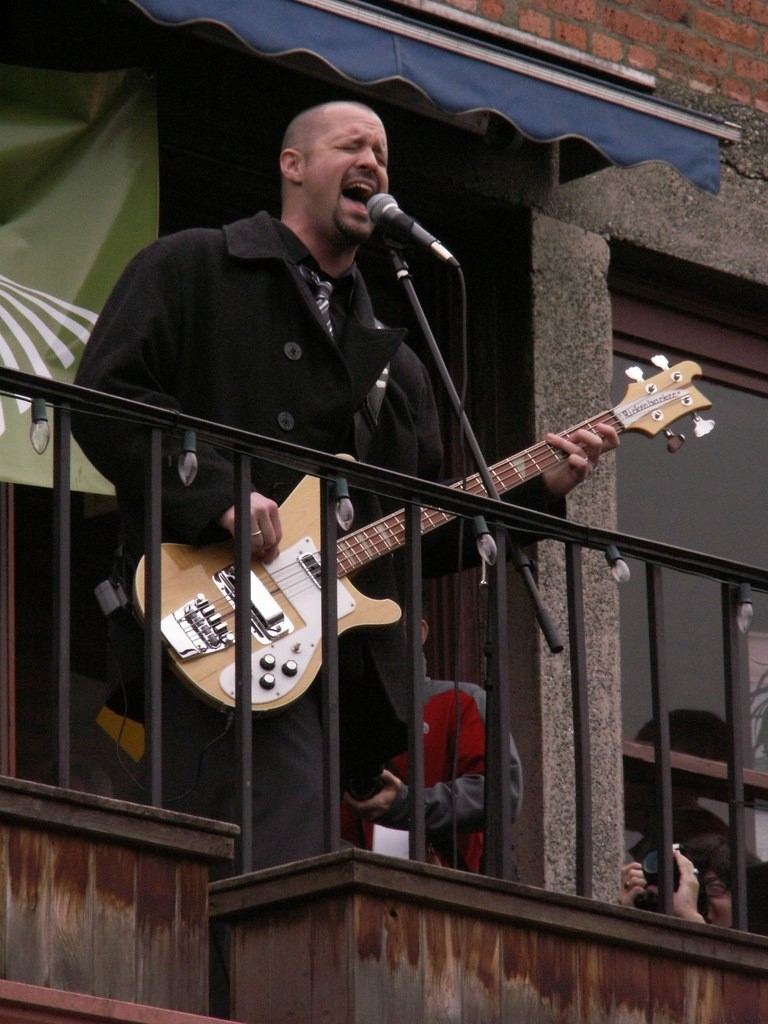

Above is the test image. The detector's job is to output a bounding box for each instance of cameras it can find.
[640,849,681,892]
[346,774,385,801]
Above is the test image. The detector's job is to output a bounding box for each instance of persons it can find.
[337,583,523,880]
[620,834,764,933]
[72,98,621,877]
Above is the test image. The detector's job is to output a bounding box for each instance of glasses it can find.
[702,878,728,897]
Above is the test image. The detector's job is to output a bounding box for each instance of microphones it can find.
[366,192,460,270]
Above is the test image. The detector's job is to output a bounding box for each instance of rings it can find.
[624,882,630,888]
[251,530,262,536]
[693,868,698,874]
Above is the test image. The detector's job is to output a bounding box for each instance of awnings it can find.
[0,0,743,195]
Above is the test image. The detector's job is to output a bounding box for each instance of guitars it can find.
[128,346,720,713]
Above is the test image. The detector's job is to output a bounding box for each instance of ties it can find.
[300,264,333,339]
